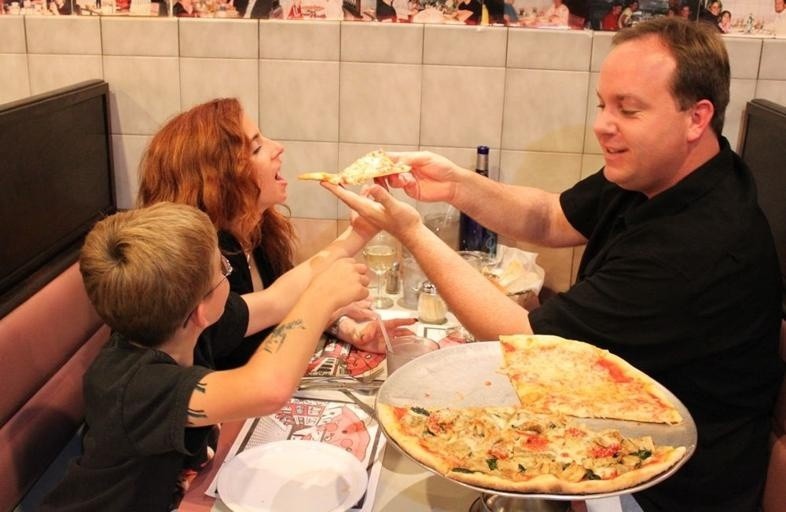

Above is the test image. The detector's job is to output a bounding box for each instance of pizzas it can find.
[297,150,413,186]
[376,333,687,494]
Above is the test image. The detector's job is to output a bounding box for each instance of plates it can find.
[215,436,371,512]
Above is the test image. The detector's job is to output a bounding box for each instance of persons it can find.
[320,19,786,512]
[0,0,281,19]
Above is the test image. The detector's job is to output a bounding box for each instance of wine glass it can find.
[361,230,400,311]
[445,247,484,343]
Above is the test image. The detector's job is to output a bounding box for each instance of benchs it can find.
[1,243,112,512]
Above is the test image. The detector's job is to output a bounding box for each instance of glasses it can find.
[182,255,234,329]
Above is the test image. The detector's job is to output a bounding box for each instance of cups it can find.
[422,210,453,243]
[385,336,442,379]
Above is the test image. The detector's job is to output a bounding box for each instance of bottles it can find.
[457,143,501,256]
[416,280,449,327]
